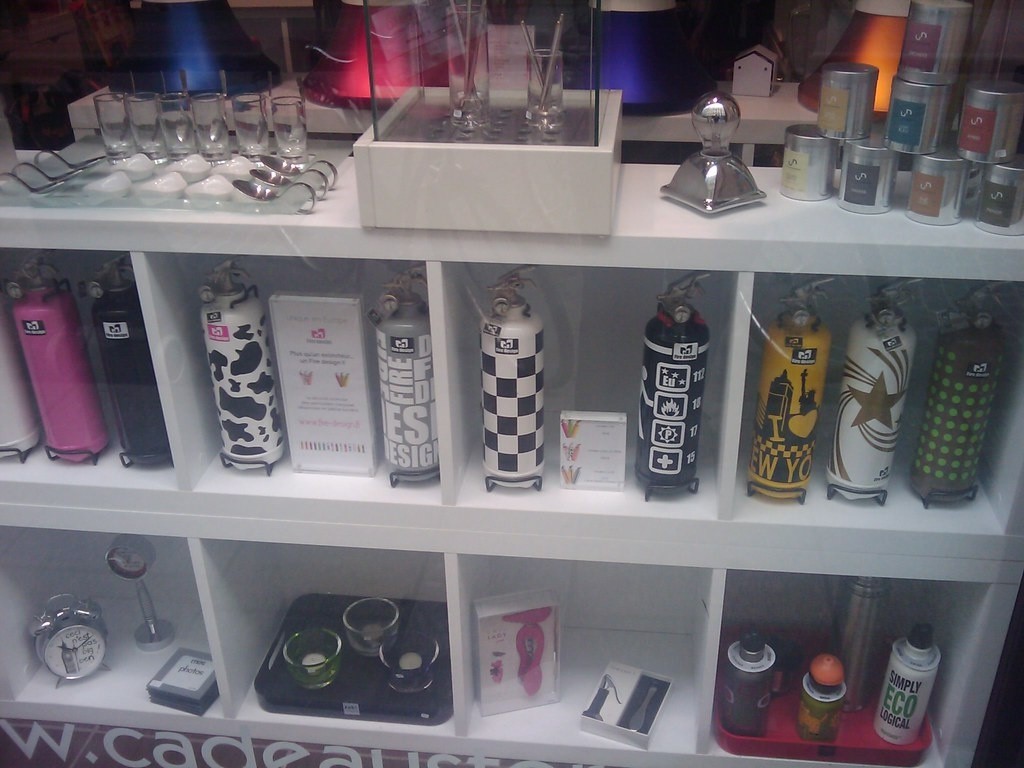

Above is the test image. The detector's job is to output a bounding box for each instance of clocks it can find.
[105,534,176,651]
[27,592,111,689]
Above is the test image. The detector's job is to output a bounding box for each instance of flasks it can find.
[836,576,889,711]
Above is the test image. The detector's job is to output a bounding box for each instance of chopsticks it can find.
[521,14,564,111]
[450,0,486,103]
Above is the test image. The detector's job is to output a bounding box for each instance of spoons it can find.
[35,150,106,170]
[261,156,338,190]
[210,70,227,141]
[11,161,88,182]
[232,180,316,215]
[176,69,193,143]
[257,71,271,144]
[249,169,328,201]
[0,172,67,193]
[290,76,307,146]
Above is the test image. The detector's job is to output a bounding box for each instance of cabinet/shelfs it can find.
[0,132,1024,768]
[67,73,816,141]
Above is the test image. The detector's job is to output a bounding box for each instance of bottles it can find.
[874,623,940,744]
[797,654,846,743]
[723,632,778,736]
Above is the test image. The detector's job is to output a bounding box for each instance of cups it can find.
[446,5,489,126]
[272,96,307,164]
[192,93,231,165]
[527,49,564,127]
[93,93,135,165]
[125,92,168,164]
[377,630,439,693]
[342,597,399,654]
[158,92,197,164]
[282,627,341,688]
[232,92,268,162]
